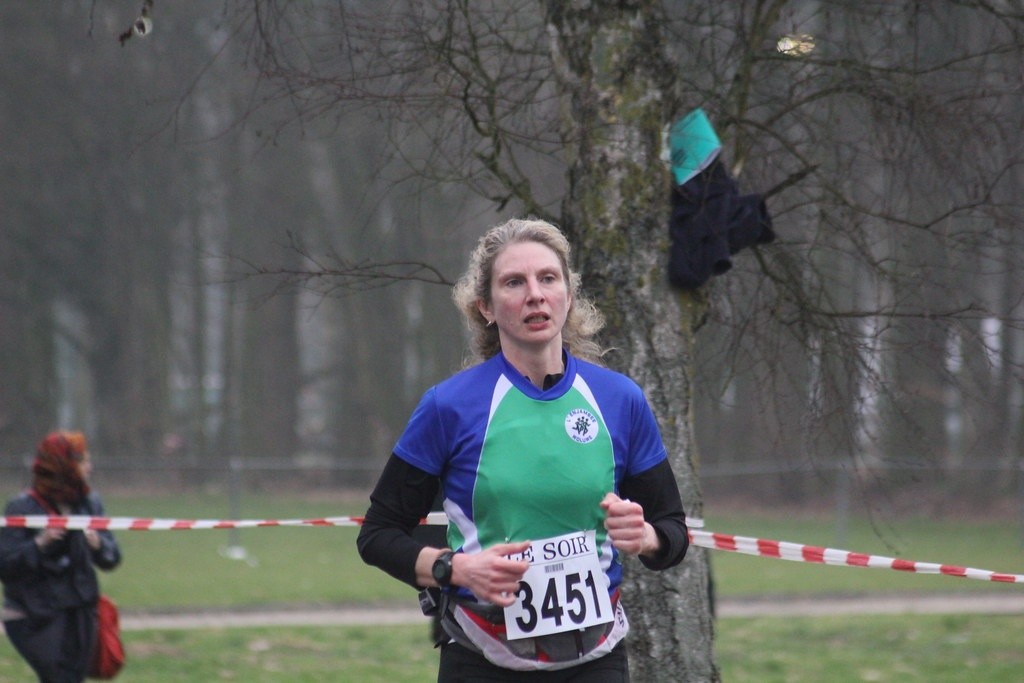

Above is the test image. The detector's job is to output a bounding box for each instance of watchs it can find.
[432,551,457,588]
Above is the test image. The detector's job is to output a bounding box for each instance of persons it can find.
[355,220,690,683]
[0,432,121,683]
